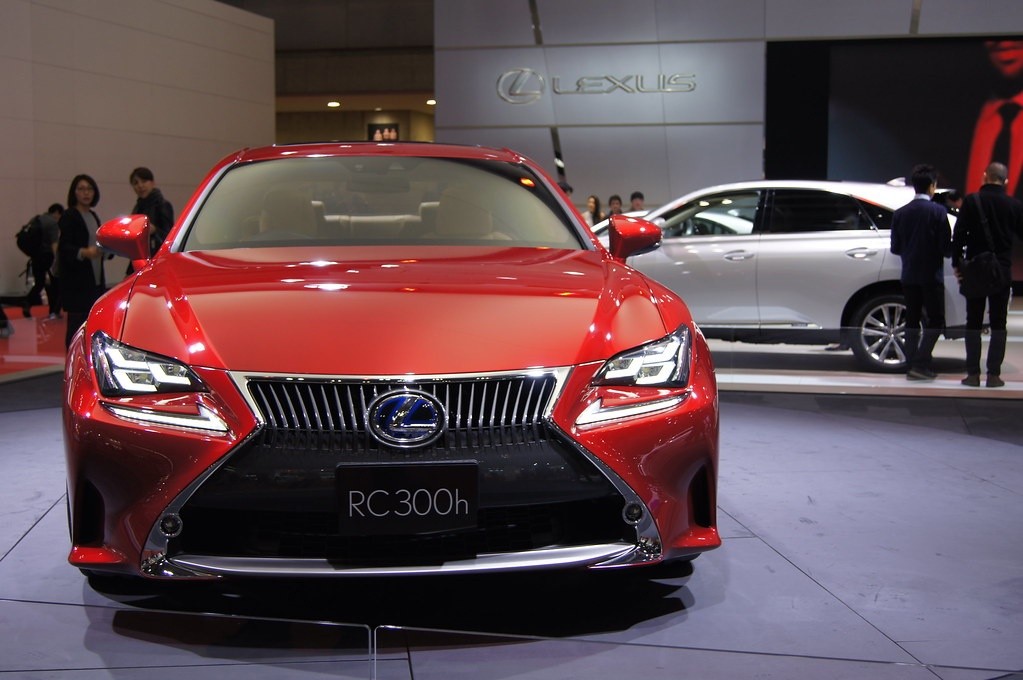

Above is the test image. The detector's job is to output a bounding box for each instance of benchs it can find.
[243,202,439,240]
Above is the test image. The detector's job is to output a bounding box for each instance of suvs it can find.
[583,178,1013,374]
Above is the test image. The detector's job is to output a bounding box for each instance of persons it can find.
[889,165,952,379]
[943,35,1023,202]
[582,194,605,228]
[602,195,623,221]
[53,174,109,349]
[629,191,644,212]
[557,182,573,199]
[125,166,173,277]
[20,203,66,322]
[952,162,1023,389]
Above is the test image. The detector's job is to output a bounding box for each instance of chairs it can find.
[238,184,319,241]
[433,177,509,241]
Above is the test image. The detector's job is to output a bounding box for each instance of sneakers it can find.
[906,367,938,380]
[961,374,981,386]
[985,371,1005,387]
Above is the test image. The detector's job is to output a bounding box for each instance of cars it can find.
[59,140,728,599]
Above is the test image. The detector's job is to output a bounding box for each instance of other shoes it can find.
[49,312,65,320]
[0,323,15,337]
[20,297,32,317]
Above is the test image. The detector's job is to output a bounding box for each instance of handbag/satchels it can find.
[954,252,1006,297]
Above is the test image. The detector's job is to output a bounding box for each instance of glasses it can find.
[75,185,95,194]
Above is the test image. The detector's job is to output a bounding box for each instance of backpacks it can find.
[15,215,46,257]
